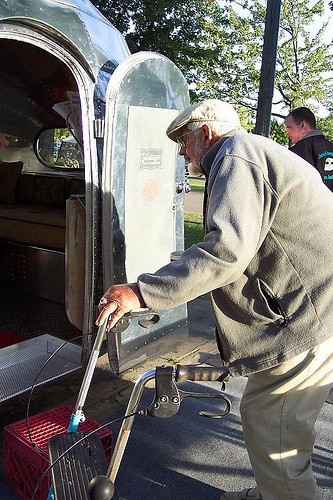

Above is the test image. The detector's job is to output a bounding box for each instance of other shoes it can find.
[219,487,263,500]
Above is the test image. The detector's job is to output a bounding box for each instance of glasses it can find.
[176,127,202,148]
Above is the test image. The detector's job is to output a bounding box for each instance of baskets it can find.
[2,402,114,500]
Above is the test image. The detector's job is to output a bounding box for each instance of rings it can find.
[99,297,108,306]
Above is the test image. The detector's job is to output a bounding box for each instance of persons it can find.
[285,106,333,194]
[95,97,332,500]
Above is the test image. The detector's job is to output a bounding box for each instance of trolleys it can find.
[25,309,234,500]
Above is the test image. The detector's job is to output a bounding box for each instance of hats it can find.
[166,99,240,143]
